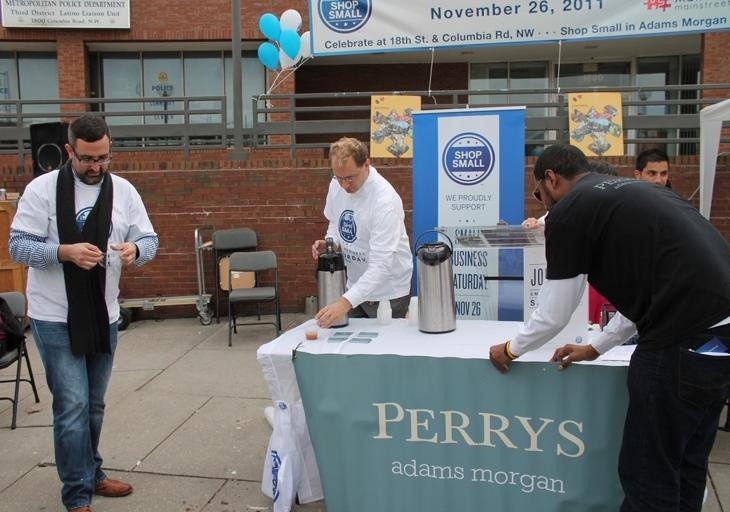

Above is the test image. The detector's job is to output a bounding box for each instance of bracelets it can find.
[504,342,512,360]
[507,341,516,359]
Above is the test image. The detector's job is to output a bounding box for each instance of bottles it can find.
[0,187,7,201]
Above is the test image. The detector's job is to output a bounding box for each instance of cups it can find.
[303,323,320,340]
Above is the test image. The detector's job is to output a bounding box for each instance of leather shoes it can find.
[68,506,91,512]
[95,478,133,497]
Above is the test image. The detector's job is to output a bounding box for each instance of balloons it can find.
[257,43,280,75]
[259,13,282,42]
[301,31,311,59]
[279,28,303,62]
[277,48,303,70]
[279,9,303,34]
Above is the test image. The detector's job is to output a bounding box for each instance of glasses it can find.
[532,169,552,201]
[331,165,363,181]
[69,144,112,165]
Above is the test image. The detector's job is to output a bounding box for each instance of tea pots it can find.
[315,237,350,328]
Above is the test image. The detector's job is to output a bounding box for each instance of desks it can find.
[260,310,669,511]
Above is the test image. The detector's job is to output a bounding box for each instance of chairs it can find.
[0,289,39,412]
[208,228,283,348]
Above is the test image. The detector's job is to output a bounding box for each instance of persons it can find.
[520,162,618,327]
[312,135,414,329]
[633,148,674,192]
[490,144,730,512]
[8,114,160,512]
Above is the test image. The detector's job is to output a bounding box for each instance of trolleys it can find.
[114,222,216,331]
